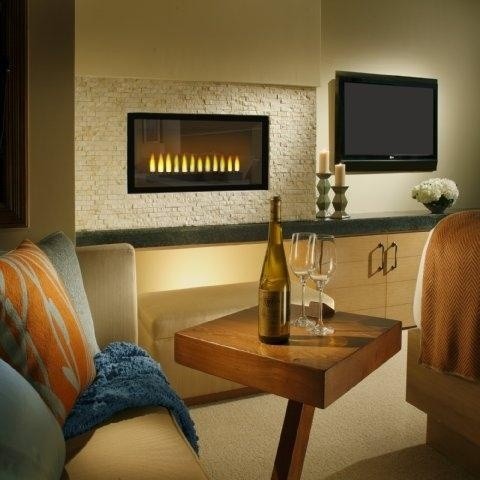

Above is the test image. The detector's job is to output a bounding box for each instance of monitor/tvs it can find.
[335,70,438,172]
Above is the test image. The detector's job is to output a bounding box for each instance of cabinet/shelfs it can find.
[321,230,429,330]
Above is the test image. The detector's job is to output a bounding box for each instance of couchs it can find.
[1,240,211,480]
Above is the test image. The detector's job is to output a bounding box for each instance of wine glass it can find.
[287,233,337,336]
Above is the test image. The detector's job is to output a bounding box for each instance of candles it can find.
[319,152,346,187]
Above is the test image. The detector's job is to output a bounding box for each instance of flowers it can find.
[411,177,459,206]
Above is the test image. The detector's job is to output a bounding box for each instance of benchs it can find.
[138,280,336,406]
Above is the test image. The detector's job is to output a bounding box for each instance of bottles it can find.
[258,195,292,345]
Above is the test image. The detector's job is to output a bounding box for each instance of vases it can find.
[422,200,453,214]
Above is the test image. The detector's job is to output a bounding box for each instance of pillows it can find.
[1,229,100,479]
